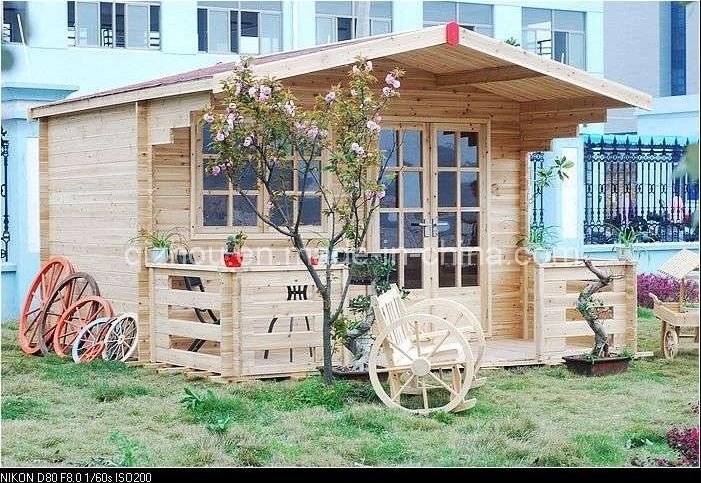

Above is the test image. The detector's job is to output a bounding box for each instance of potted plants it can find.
[601,223,662,261]
[562,257,635,377]
[126,222,192,265]
[309,232,338,265]
[223,231,248,267]
[525,224,560,265]
[315,255,412,382]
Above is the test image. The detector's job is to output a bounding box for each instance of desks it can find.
[262,285,313,362]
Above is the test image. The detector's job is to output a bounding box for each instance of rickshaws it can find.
[648,292,698,359]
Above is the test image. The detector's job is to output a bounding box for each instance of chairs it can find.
[175,253,221,352]
[441,221,476,247]
[367,282,487,419]
